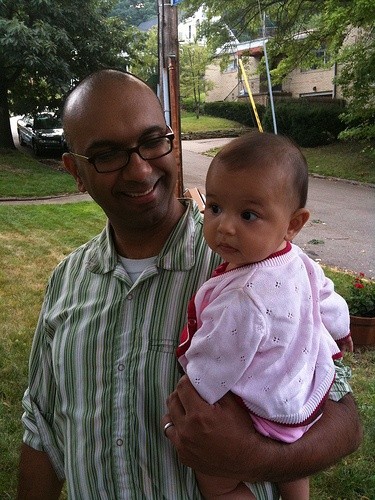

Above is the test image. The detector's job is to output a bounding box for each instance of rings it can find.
[163,422,174,438]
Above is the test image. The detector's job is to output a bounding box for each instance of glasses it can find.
[69,125,175,174]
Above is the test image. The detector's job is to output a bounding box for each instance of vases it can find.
[350,314,375,346]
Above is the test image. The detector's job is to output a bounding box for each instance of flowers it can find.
[343,272,375,314]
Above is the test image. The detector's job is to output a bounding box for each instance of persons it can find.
[16,69,364,500]
[176,132,354,500]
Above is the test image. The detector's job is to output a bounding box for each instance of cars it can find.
[16,112,68,157]
